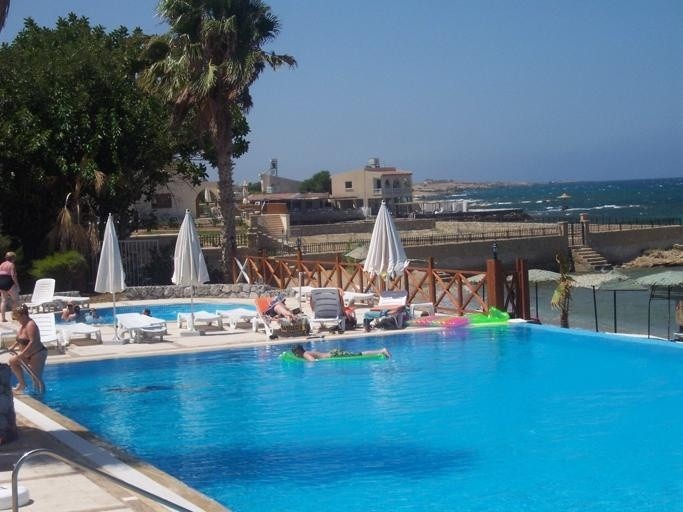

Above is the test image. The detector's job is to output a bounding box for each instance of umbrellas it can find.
[362,199,411,296]
[345,247,367,261]
[94,212,127,338]
[172,209,210,330]
[463,269,683,340]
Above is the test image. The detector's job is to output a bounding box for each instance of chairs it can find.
[2,278,102,350]
[255,287,434,340]
[177,311,224,332]
[114,313,167,344]
[217,307,260,332]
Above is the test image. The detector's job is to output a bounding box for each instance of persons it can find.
[268,292,294,321]
[142,308,152,316]
[8,304,48,391]
[61,300,104,325]
[1,251,21,322]
[291,343,390,364]
